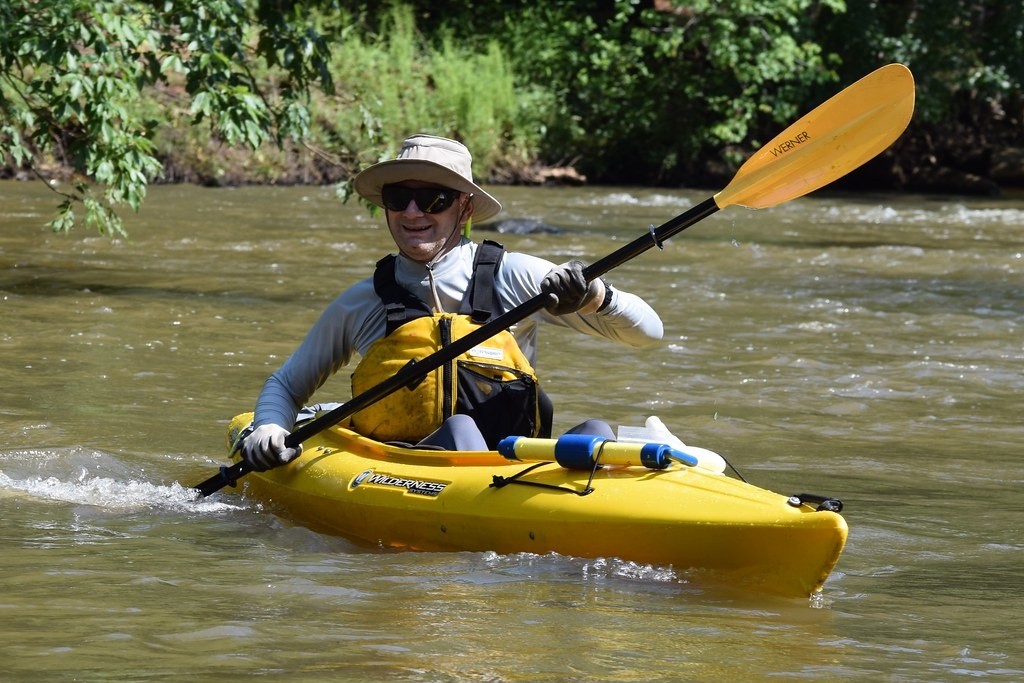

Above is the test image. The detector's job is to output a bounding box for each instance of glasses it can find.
[382,183,461,214]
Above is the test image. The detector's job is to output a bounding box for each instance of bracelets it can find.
[596,278,613,313]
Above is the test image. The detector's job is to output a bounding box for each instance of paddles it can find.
[190,61,917,499]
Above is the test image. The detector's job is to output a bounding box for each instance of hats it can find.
[354,133,503,225]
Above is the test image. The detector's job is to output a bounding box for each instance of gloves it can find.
[540,260,599,316]
[240,423,304,473]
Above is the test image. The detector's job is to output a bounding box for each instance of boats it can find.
[226,402,849,599]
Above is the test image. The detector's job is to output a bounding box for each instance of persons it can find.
[240,133,664,473]
